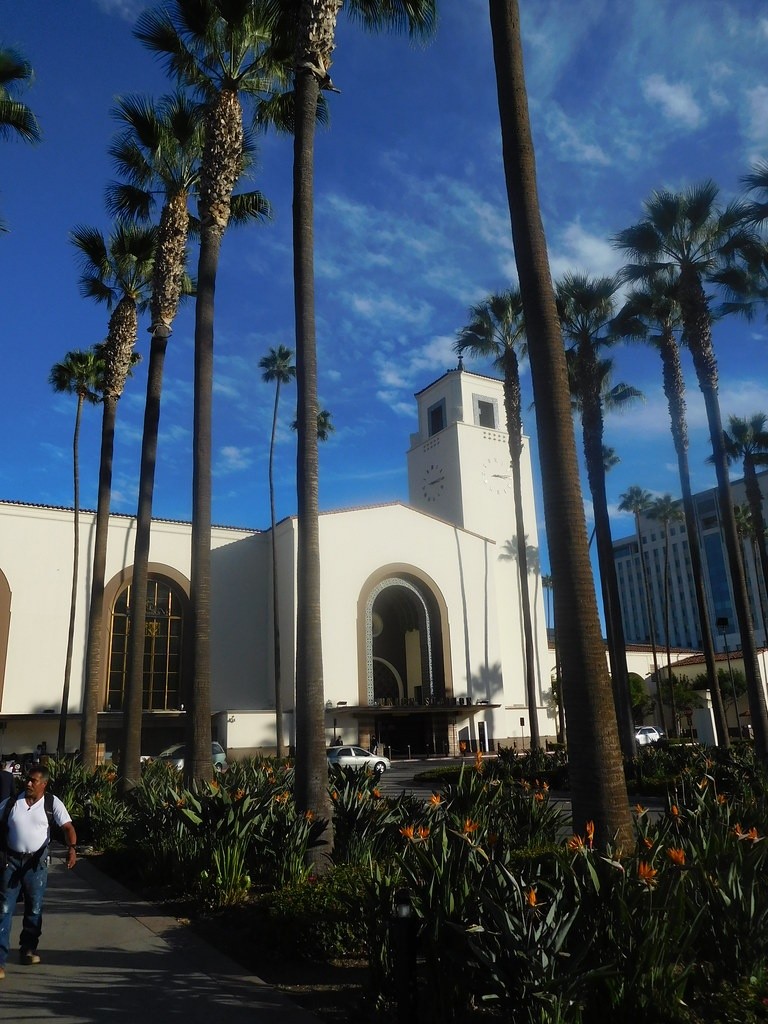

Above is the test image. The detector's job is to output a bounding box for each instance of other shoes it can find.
[0,965,5,979]
[20,950,40,964]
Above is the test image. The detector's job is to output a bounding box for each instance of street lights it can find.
[717,625,743,740]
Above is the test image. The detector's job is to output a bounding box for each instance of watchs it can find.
[70,844,77,850]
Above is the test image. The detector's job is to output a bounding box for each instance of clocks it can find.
[422,465,445,502]
[482,458,513,495]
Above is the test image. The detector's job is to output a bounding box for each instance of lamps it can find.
[180,704,185,710]
[107,704,111,712]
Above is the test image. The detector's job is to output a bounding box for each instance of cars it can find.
[148,742,229,776]
[635,727,661,745]
[326,745,391,776]
[2,754,41,778]
[654,727,667,738]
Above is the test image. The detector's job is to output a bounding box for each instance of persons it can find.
[335,736,343,746]
[0,764,76,978]
[33,744,41,765]
[371,735,378,754]
[0,761,16,803]
